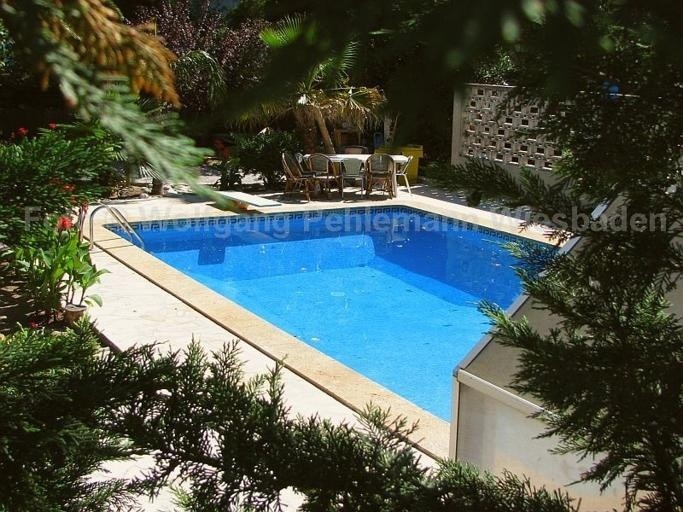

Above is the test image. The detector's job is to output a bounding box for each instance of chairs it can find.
[282,145,414,202]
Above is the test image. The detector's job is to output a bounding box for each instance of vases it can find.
[28,304,87,330]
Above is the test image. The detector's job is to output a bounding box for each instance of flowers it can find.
[15,213,113,321]
